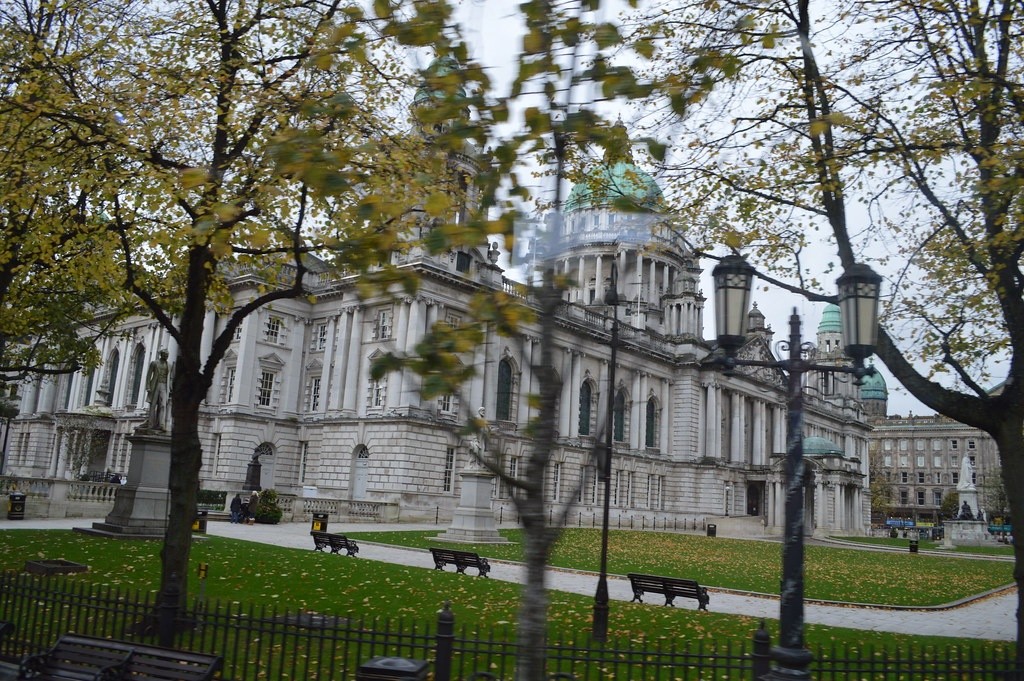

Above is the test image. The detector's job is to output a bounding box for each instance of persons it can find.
[958,453,973,488]
[230,490,259,525]
[951,500,984,521]
[146,348,174,431]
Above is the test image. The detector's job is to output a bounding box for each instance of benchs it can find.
[627,572,710,611]
[428,545,490,578]
[311,529,359,558]
[17,633,224,681]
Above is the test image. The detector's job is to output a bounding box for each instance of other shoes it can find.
[235,522,237,524]
[231,521,233,523]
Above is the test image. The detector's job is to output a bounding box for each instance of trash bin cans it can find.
[707,524,716,536]
[6,494,26,519]
[311,512,328,534]
[192,508,207,534]
[909,538,919,555]
